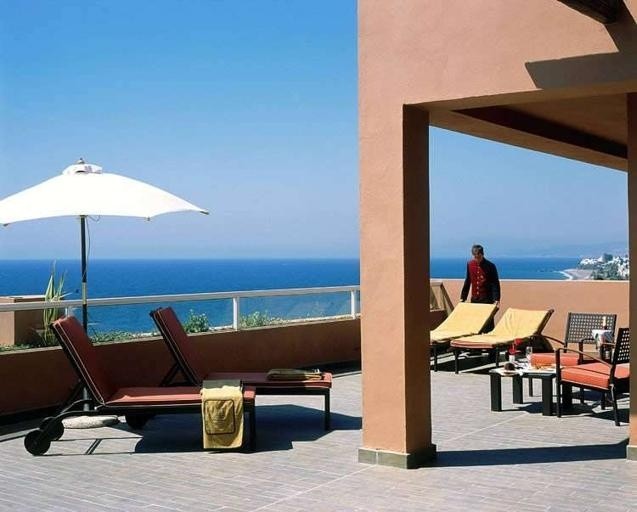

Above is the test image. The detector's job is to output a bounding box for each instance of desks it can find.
[484,365,555,417]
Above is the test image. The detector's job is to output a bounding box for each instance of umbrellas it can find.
[0,157,211,411]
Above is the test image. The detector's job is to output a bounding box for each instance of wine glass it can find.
[525,346,532,371]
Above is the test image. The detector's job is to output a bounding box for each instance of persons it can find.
[460,245,500,355]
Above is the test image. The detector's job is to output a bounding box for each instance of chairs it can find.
[555,326,632,430]
[23,313,258,456]
[449,307,556,377]
[150,306,333,434]
[429,300,499,372]
[528,311,618,398]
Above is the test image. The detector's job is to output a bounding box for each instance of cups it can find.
[591,329,612,350]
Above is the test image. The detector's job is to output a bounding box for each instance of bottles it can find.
[599,316,611,364]
[508,341,514,364]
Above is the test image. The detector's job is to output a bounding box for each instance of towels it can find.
[452,303,551,348]
[200,378,244,452]
[265,364,326,384]
[429,301,497,345]
